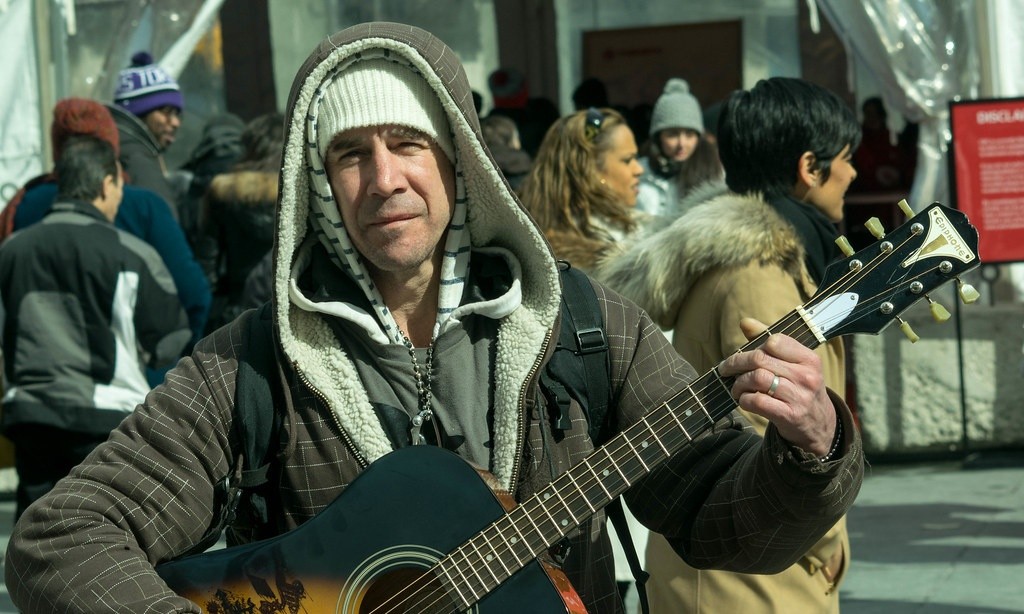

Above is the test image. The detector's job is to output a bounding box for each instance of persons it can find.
[591,76,859,614]
[0,52,918,521]
[514,105,674,601]
[5,22,863,614]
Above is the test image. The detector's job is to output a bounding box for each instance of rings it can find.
[767,374,779,395]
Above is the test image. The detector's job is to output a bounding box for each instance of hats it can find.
[49,97,120,163]
[111,51,183,117]
[316,60,457,164]
[203,113,244,132]
[649,78,704,138]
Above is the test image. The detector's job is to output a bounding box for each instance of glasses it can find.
[584,107,603,138]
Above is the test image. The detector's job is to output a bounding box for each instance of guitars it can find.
[151,197,982,614]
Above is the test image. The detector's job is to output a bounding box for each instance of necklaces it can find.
[396,322,435,447]
